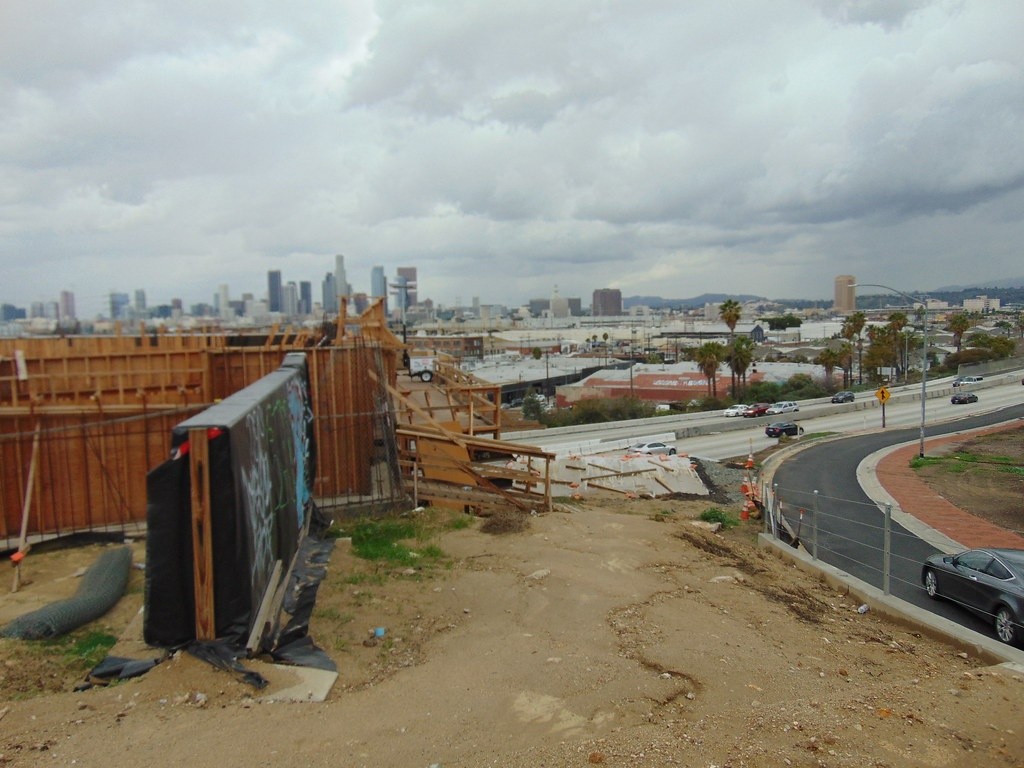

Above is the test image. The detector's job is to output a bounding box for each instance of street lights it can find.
[846,282,930,457]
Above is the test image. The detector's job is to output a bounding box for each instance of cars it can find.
[951,375,984,387]
[628,441,679,457]
[921,545,1024,646]
[951,392,978,405]
[722,390,856,441]
